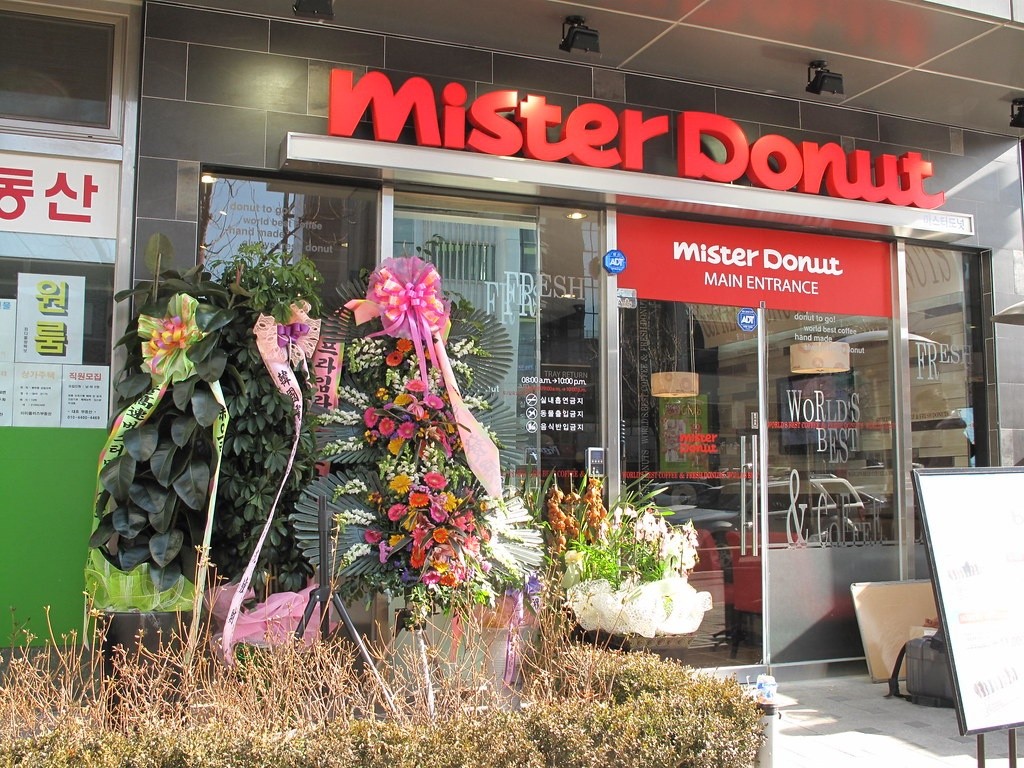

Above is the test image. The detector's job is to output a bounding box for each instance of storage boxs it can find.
[905,625,956,709]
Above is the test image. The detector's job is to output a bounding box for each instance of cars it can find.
[640,461,923,582]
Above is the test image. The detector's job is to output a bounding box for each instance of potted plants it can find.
[78,229,324,741]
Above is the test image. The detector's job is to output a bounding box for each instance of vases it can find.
[561,622,697,652]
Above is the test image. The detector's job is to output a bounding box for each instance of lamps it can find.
[806,60,844,95]
[559,16,600,55]
[790,311,851,374]
[753,673,780,715]
[293,0,335,21]
[1009,97,1024,128]
[651,299,699,397]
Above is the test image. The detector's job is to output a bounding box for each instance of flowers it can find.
[317,326,540,620]
[549,465,707,585]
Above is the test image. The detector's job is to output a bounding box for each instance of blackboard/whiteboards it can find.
[911,466,1024,738]
